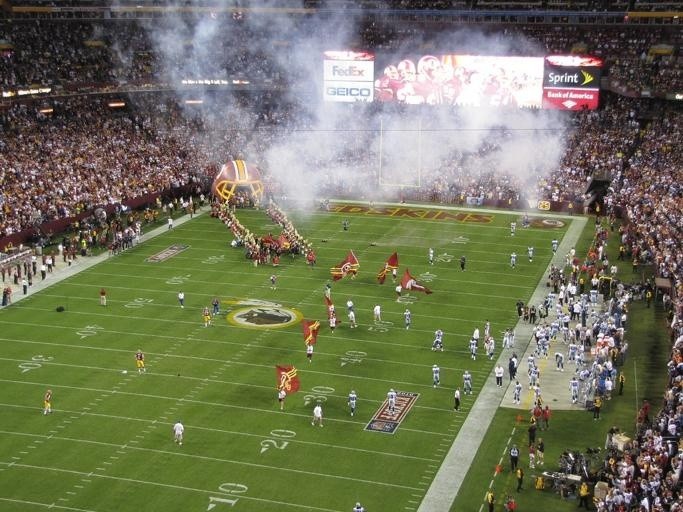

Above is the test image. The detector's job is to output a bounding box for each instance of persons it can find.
[211,297,218,315]
[373,303,381,322]
[42,389,52,415]
[0,0,682,311]
[418,233,681,512]
[347,390,356,417]
[349,309,357,329]
[200,306,210,328]
[277,388,285,410]
[403,309,410,331]
[352,502,364,512]
[305,342,313,362]
[385,388,396,417]
[173,419,183,445]
[327,310,336,334]
[310,403,323,428]
[134,349,145,375]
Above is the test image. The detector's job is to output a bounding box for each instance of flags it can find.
[275,364,299,396]
[302,318,320,347]
